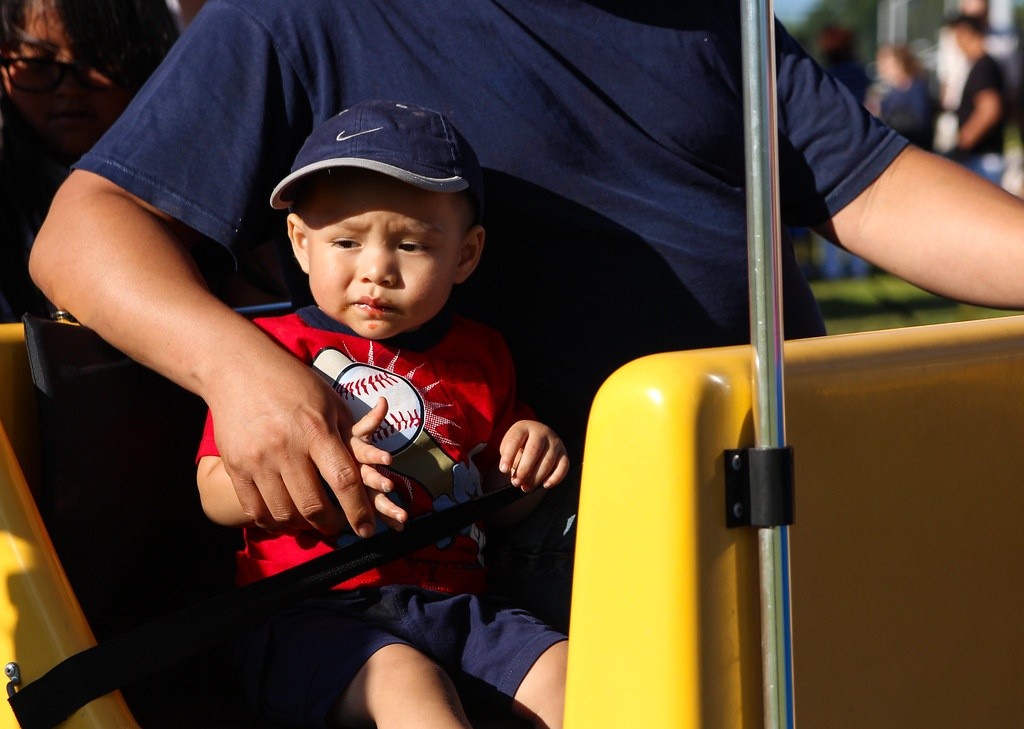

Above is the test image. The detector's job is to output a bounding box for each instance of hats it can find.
[270,99,483,210]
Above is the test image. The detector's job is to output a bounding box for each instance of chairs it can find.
[1,318,315,729]
[566,306,1021,729]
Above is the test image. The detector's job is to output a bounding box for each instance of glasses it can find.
[0,54,119,93]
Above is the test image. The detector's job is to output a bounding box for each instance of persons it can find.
[942,16,1009,186]
[0,0,180,323]
[874,42,936,150]
[808,21,872,276]
[193,102,568,729]
[934,0,1012,152]
[27,1,1024,640]
